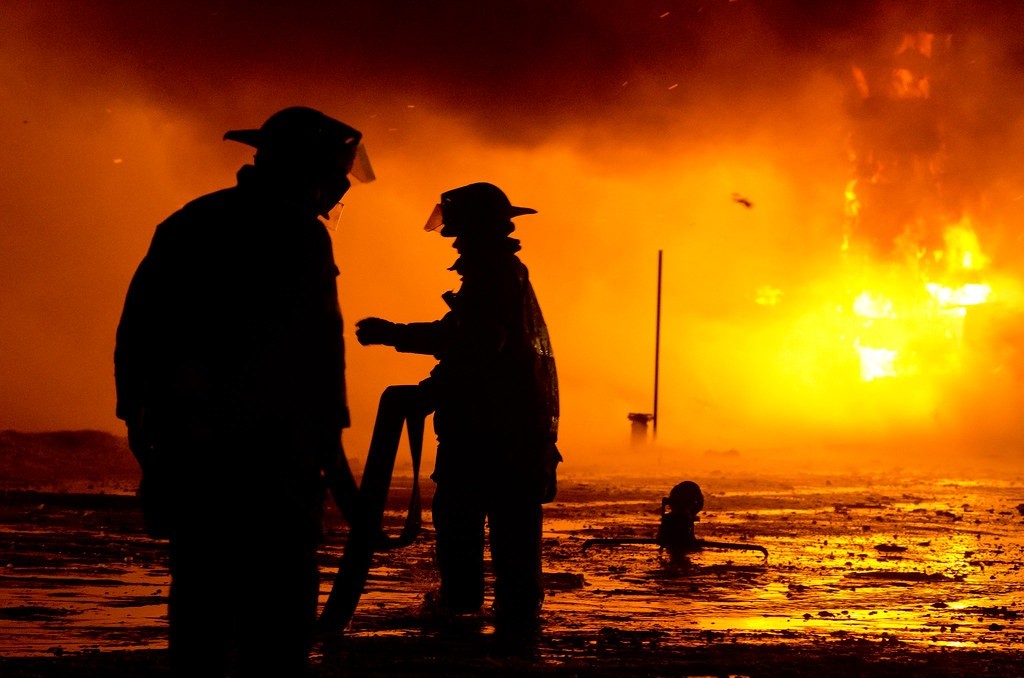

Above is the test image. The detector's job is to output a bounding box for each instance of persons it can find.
[114,105,377,678]
[354,182,563,626]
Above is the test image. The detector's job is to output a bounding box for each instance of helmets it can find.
[424,183,537,231]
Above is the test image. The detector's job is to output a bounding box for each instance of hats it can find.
[223,107,376,183]
